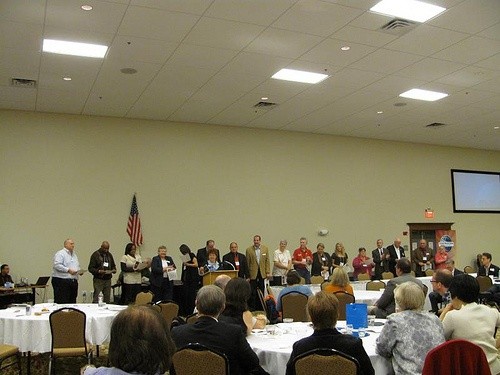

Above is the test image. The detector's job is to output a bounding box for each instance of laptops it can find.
[31,277,50,286]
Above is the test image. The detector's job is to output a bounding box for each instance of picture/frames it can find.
[451,168,500,213]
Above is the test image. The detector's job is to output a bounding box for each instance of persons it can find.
[179,244,198,267]
[197,240,221,268]
[200,249,222,274]
[332,242,348,273]
[353,238,449,281]
[311,242,331,277]
[324,267,353,297]
[120,243,150,305]
[291,237,314,284]
[151,245,177,302]
[0,264,14,286]
[51,238,83,304]
[85,252,500,375]
[222,241,250,282]
[272,239,291,285]
[88,241,117,304]
[246,235,270,291]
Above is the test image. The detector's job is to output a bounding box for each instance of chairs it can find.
[135,291,153,304]
[170,343,230,375]
[156,300,179,330]
[292,348,362,375]
[110,284,122,302]
[0,340,22,375]
[48,308,93,375]
[257,266,493,323]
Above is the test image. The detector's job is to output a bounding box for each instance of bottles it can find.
[4,281,15,294]
[98,291,103,308]
[26,305,31,316]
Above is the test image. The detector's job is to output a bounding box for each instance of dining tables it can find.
[246,320,394,375]
[313,290,385,307]
[0,301,128,375]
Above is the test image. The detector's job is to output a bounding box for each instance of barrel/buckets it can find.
[346,304,367,329]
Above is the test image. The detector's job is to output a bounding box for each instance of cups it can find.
[346,324,353,334]
[101,303,106,308]
[266,325,275,336]
[283,318,293,332]
[47,298,54,306]
[358,328,365,337]
[367,315,375,328]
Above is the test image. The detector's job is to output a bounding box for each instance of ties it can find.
[380,249,382,255]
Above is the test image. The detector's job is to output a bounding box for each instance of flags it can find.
[127,195,143,246]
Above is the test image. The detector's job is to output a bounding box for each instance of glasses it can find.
[430,280,443,283]
